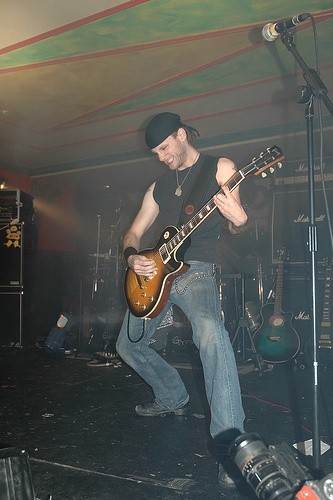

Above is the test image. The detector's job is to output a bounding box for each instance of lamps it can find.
[239,302,262,333]
[45,312,75,351]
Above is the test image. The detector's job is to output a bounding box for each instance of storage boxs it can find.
[0,188,33,348]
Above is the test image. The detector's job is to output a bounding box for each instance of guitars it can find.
[123,146,286,320]
[304,255,333,365]
[251,246,301,364]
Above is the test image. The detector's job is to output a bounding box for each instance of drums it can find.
[210,274,244,318]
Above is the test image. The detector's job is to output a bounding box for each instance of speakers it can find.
[271,186,333,264]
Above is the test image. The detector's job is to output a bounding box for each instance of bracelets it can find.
[124,247,137,262]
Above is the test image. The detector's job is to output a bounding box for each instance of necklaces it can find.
[175,153,199,197]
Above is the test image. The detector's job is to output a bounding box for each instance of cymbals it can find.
[90,253,116,259]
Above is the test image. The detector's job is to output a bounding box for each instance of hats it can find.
[144,112,183,150]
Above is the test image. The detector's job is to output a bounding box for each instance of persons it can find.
[115,112,249,488]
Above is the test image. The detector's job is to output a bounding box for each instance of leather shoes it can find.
[134,398,189,416]
[217,454,245,488]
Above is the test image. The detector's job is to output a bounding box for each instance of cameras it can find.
[226,436,333,500]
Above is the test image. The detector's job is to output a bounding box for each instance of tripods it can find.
[230,270,262,370]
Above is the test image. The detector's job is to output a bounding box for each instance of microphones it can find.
[261,12,310,41]
